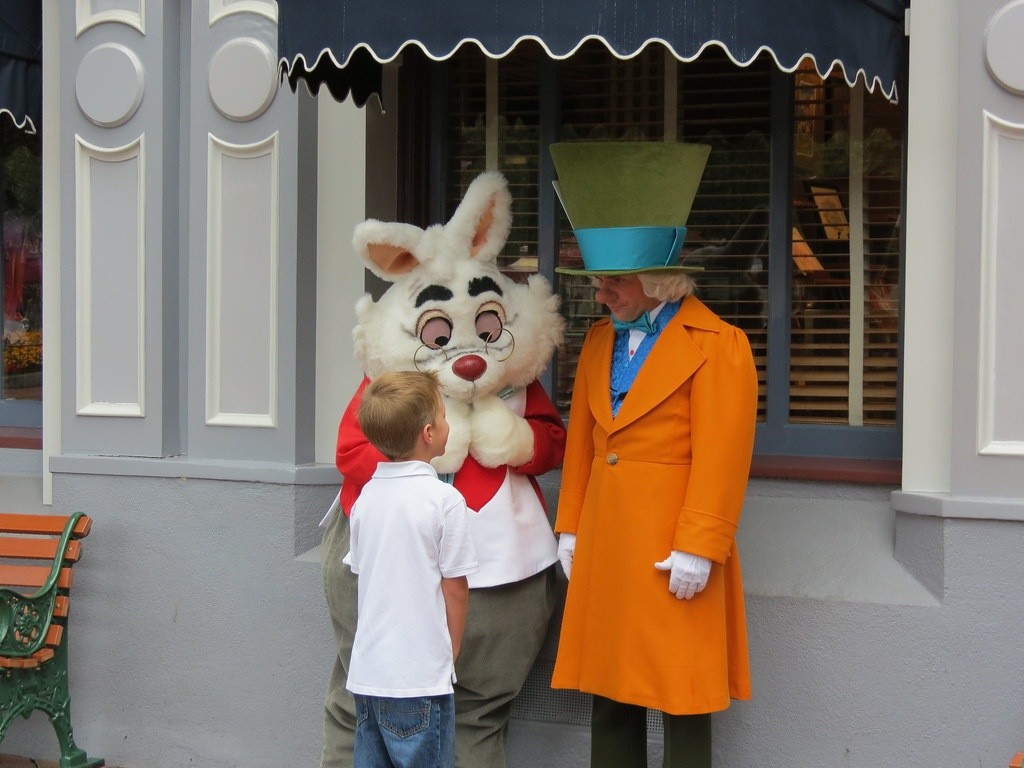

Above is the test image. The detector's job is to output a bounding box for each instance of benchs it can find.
[0,511,106,768]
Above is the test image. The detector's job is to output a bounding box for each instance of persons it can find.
[341,370,477,768]
[549,223,759,768]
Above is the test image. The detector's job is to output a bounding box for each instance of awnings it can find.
[279,1,909,114]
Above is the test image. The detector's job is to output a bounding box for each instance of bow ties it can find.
[611,311,656,335]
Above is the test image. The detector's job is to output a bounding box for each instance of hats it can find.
[547,140,712,276]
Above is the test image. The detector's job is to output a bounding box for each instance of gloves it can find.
[654,550,712,601]
[557,533,576,581]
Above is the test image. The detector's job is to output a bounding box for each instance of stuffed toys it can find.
[321,170,569,768]
